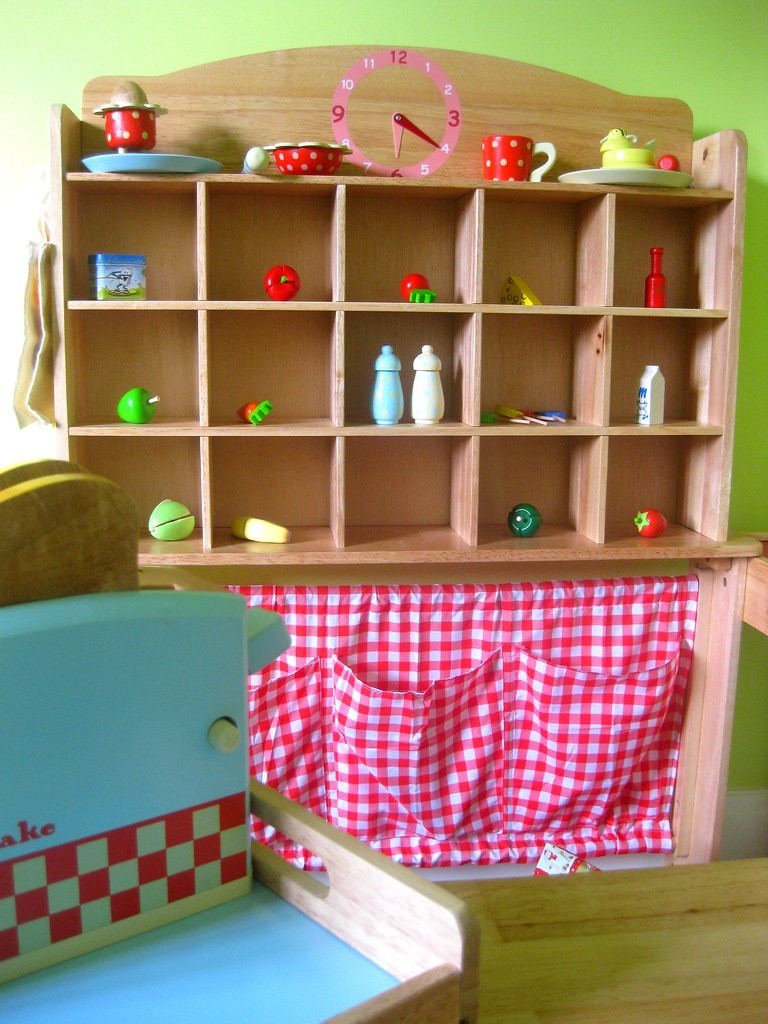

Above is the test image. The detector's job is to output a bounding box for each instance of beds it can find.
[0,589,482,1024]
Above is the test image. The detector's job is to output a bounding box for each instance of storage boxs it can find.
[87,253,148,301]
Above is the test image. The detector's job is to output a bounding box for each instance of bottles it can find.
[645,248,667,307]
[411,345,445,424]
[370,344,404,424]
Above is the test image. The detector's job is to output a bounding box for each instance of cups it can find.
[481,135,557,181]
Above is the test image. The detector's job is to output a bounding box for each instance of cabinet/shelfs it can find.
[50,46,763,570]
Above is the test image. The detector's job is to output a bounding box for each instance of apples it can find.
[116,387,160,424]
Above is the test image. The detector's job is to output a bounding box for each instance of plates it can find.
[81,154,224,172]
[558,167,692,189]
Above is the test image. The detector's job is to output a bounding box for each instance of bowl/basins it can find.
[263,142,353,176]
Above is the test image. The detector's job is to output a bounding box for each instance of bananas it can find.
[229,516,292,545]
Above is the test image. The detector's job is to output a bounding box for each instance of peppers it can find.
[634,509,668,538]
[508,503,541,537]
[264,265,300,301]
